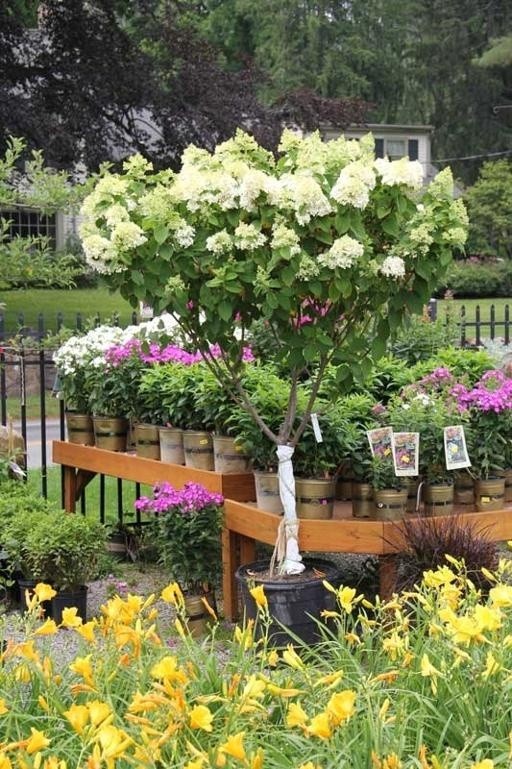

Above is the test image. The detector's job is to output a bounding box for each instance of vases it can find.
[171,583,216,638]
[232,558,343,669]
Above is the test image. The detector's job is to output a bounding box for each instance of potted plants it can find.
[0,447,125,631]
